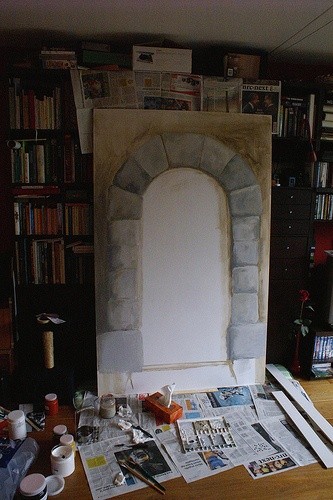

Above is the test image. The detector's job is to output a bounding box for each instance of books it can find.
[14,237,95,286]
[312,335,333,360]
[38,41,131,70]
[277,94,333,140]
[9,133,94,186]
[8,76,64,130]
[315,194,333,220]
[303,161,329,189]
[11,185,94,236]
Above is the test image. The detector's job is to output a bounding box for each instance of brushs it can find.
[126,419,154,438]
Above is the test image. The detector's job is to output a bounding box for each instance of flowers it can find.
[294,289,315,336]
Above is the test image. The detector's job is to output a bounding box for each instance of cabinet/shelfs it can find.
[0,67,333,375]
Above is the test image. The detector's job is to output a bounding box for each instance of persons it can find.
[243,92,260,114]
[262,93,277,122]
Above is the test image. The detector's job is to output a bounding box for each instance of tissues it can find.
[143,382,183,424]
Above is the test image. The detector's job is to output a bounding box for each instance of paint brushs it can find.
[127,456,166,491]
[116,458,165,495]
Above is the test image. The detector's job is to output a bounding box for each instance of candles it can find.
[43,332,55,368]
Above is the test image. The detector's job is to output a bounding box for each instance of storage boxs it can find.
[224,53,260,79]
[80,40,192,72]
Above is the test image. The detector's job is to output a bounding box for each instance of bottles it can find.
[101,393,116,419]
[51,443,75,478]
[6,410,26,440]
[45,394,59,416]
[52,425,68,445]
[19,473,48,500]
[59,434,76,458]
